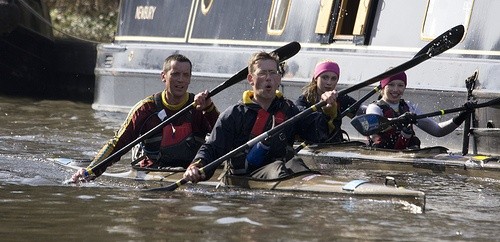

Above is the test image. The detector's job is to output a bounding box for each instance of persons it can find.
[71,54,218,186]
[293,59,367,145]
[365,67,478,151]
[183,51,338,183]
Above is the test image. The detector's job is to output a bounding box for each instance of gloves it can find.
[453,102,476,127]
[399,112,416,134]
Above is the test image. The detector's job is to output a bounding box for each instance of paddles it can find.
[146,23,464,192]
[67,39,302,183]
[349,96,500,136]
[294,85,381,155]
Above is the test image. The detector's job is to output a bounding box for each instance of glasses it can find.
[251,71,280,78]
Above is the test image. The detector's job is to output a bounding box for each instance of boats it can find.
[91,0,500,157]
[45,143,426,213]
[292,140,500,183]
